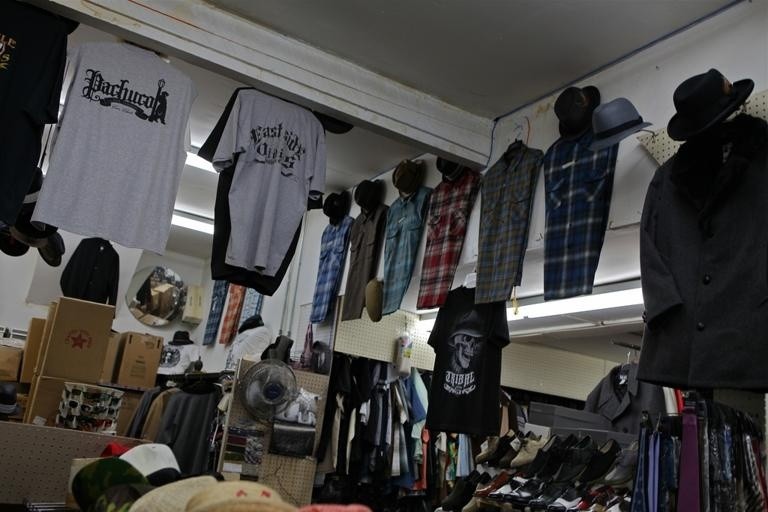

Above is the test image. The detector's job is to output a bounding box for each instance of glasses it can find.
[55,388,118,431]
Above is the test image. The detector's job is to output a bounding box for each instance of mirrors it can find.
[125,265,186,326]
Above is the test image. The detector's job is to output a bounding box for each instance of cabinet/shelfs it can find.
[182,286,204,323]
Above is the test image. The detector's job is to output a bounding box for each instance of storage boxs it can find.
[151,284,174,313]
[0,296,165,437]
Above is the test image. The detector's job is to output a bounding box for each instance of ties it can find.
[630,416,680,512]
[676,394,768,512]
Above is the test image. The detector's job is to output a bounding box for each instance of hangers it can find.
[463,254,478,287]
[159,371,217,393]
[504,125,526,156]
[615,351,637,377]
[639,402,761,434]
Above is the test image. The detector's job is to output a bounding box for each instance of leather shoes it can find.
[583,439,620,484]
[502,465,553,501]
[564,484,613,512]
[554,435,598,484]
[604,489,634,512]
[488,471,529,498]
[529,434,565,480]
[547,479,588,510]
[441,470,479,510]
[474,471,508,498]
[488,430,516,467]
[541,433,578,480]
[460,472,496,512]
[511,477,551,505]
[526,476,572,508]
[498,431,525,469]
[591,487,628,511]
[474,435,499,465]
[604,439,638,486]
[510,432,551,469]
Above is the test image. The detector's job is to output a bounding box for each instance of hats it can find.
[667,69,756,142]
[1,167,65,267]
[392,158,424,200]
[355,180,381,215]
[71,442,298,512]
[586,96,652,152]
[436,156,469,185]
[323,190,348,225]
[552,86,601,141]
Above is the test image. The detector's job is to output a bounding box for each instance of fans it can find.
[240,359,297,419]
